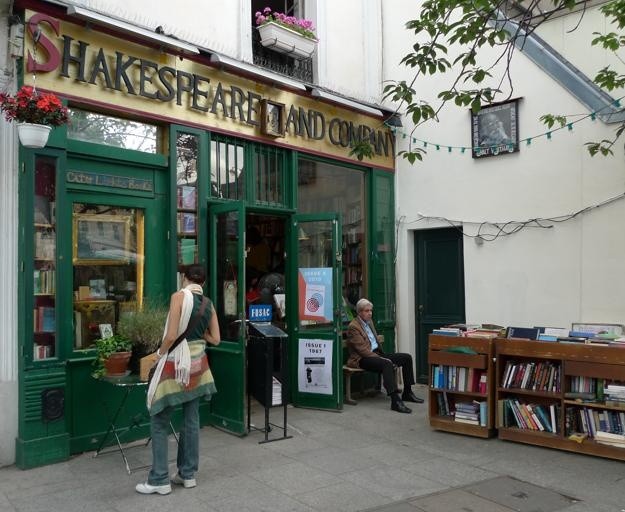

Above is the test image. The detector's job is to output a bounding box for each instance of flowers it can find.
[0,87,72,129]
[256,7,321,43]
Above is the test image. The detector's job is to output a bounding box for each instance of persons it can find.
[134,262,221,496]
[480,112,511,145]
[245,266,264,315]
[345,297,425,415]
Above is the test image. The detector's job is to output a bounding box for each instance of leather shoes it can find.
[402,392,424,403]
[391,400,411,413]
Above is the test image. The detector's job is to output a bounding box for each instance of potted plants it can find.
[91,334,132,379]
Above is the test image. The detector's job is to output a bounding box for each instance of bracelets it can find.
[156,348,163,357]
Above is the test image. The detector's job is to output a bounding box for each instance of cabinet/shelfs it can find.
[561,342,625,461]
[495,337,561,450]
[428,333,495,438]
[33,186,56,359]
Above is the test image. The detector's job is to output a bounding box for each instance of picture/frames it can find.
[470,96,524,157]
[72,213,131,266]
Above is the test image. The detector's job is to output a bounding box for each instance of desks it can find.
[93,373,179,475]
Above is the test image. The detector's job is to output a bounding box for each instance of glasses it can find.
[360,309,374,312]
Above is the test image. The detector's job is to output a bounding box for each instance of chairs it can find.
[339,335,402,405]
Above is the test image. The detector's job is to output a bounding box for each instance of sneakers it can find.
[136,480,172,495]
[170,471,197,488]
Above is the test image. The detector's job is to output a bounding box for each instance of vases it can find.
[17,122,51,149]
[254,22,318,60]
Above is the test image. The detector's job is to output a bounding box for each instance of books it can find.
[249,218,287,236]
[295,192,362,307]
[272,293,288,319]
[29,191,56,360]
[177,183,197,290]
[71,265,138,351]
[430,322,625,449]
